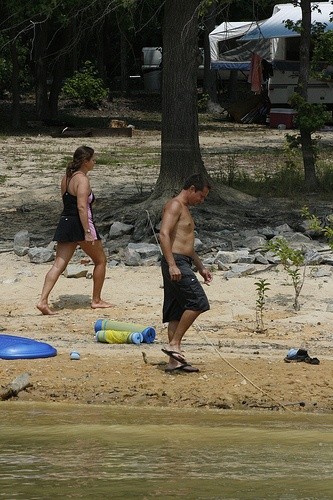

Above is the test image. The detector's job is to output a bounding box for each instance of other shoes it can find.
[284,348,310,363]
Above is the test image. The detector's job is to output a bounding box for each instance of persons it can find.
[37,146,118,315]
[160,173,213,371]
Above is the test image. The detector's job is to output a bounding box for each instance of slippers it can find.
[160,347,189,364]
[166,363,199,373]
